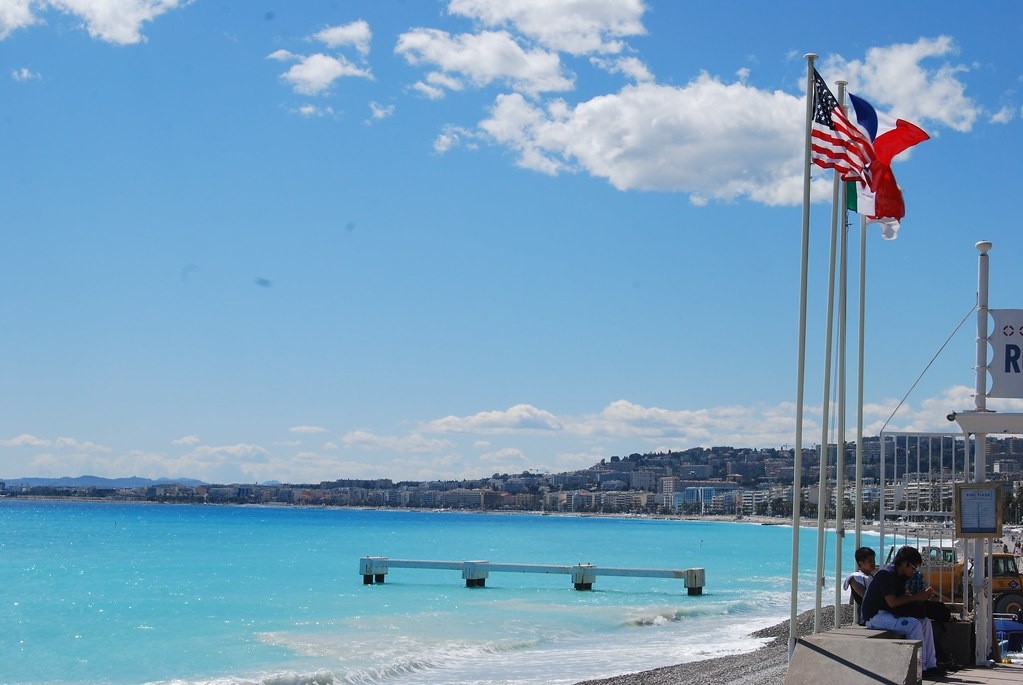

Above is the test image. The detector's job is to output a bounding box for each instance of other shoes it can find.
[922,667,947,679]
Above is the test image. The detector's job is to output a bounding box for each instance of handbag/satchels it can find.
[893,602,925,619]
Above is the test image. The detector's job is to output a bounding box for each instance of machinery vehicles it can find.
[954,552,1023,618]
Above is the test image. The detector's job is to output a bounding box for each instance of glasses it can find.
[909,562,917,570]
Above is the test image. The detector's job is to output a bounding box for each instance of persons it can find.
[843,547,965,672]
[859,546,947,680]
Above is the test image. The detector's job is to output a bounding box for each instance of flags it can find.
[810,68,877,193]
[846,90,931,241]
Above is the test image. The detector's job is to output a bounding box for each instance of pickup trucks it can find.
[871,546,966,597]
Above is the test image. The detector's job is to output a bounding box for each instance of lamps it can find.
[948,410,957,422]
